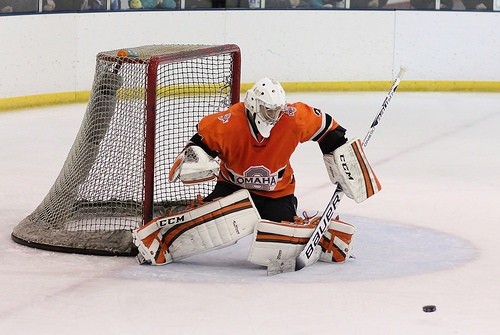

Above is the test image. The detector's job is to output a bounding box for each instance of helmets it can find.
[244,78,296,138]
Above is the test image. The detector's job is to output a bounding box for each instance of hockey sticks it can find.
[267,64,409,277]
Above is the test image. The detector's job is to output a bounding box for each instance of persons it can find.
[0,0,500,13]
[183,76,346,225]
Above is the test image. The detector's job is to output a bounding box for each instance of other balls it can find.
[423,305,436,312]
